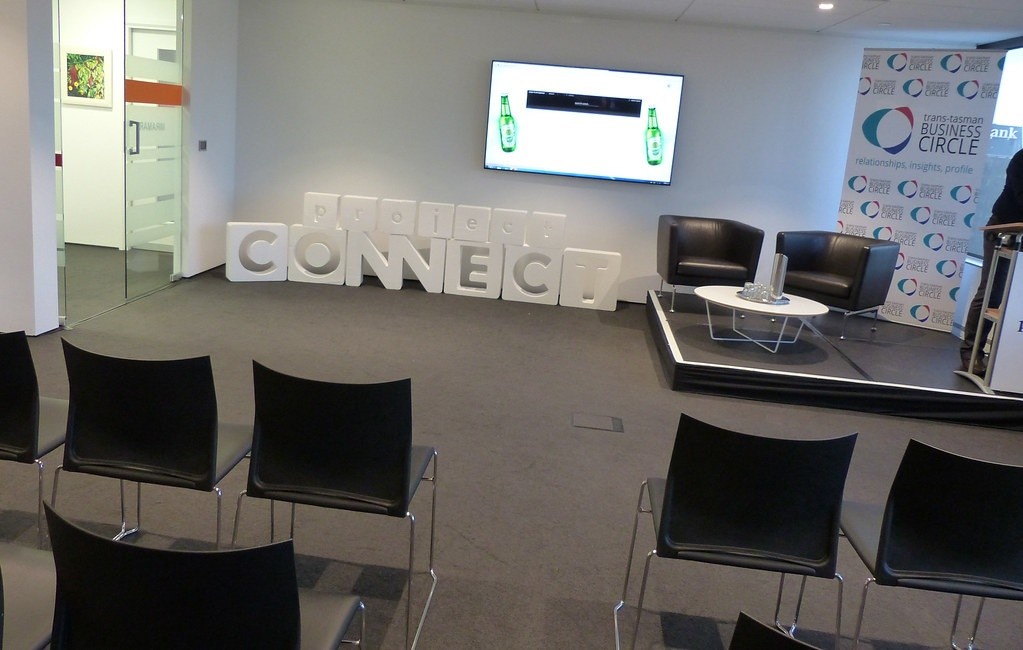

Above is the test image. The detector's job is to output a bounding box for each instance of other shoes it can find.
[962,358,986,376]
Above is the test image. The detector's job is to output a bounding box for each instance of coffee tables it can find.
[694,285,829,354]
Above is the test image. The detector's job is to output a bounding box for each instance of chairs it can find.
[0,330,69,533]
[50,336,274,552]
[614,413,859,650]
[795,439,1023,650]
[229,359,437,650]
[43,500,301,650]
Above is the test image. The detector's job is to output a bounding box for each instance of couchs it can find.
[776,231,901,339]
[656,215,765,312]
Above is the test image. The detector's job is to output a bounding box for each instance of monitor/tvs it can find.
[481,60,683,185]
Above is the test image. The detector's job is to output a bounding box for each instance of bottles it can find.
[498,95,516,152]
[645,107,664,165]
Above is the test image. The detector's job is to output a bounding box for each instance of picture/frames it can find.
[60,46,114,108]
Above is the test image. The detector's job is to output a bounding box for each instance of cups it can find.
[743,281,772,302]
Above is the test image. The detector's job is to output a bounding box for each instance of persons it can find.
[960,148,1023,374]
